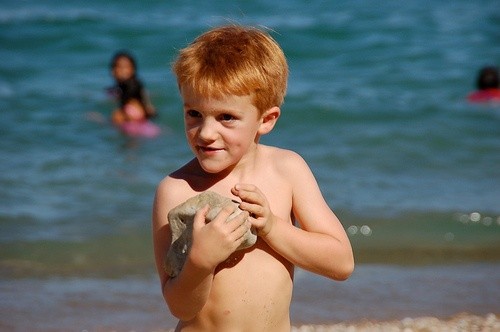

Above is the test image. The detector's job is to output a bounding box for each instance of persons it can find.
[465,66,500,103]
[103,51,155,116]
[121,93,144,120]
[153,24,358,332]
